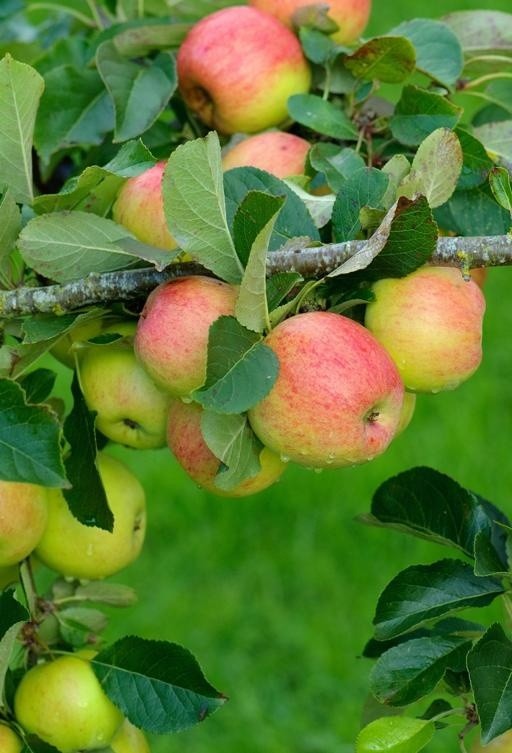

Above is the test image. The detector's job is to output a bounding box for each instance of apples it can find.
[0,648,152,753]
[472,728,512,753]
[0,0,487,582]
